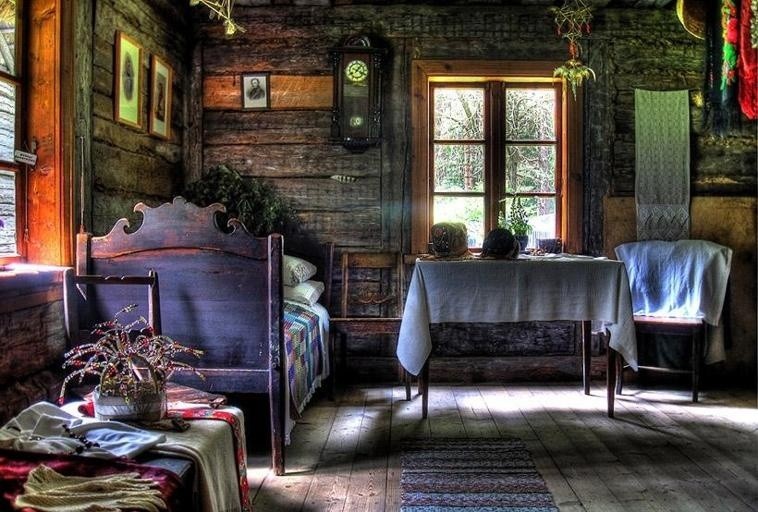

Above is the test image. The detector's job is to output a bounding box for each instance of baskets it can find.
[92,355,166,422]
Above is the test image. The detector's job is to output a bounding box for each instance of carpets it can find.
[399,436,560,512]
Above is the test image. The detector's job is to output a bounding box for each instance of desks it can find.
[405,254,626,418]
[2,391,244,512]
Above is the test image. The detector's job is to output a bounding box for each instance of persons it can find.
[155,81,166,121]
[245,78,266,100]
[122,57,133,98]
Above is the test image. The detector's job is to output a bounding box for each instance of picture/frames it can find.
[241,71,271,112]
[112,30,175,141]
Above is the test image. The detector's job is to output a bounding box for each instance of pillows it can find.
[282,253,326,308]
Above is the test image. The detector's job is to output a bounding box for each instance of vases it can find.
[90,387,167,422]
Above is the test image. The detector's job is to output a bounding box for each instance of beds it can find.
[69,195,336,477]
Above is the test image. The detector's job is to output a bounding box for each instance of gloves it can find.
[15,464,167,512]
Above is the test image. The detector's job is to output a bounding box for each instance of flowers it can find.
[53,302,208,407]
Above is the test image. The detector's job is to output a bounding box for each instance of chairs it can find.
[327,252,411,403]
[615,239,729,404]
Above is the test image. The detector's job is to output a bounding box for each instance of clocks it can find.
[329,33,389,156]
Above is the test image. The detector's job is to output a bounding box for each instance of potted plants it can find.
[512,220,529,251]
